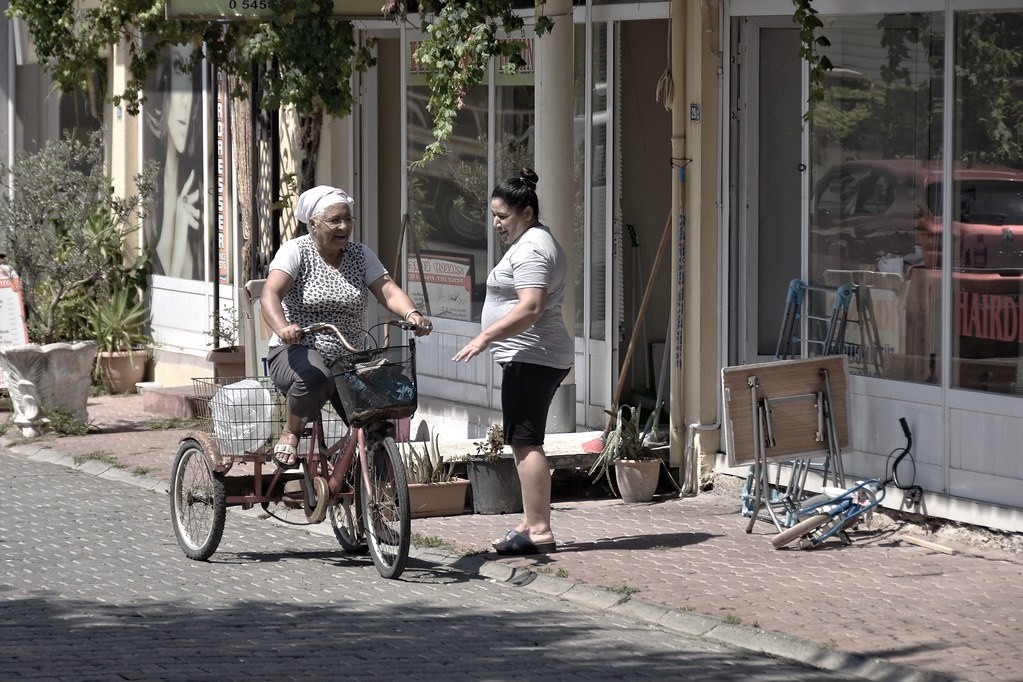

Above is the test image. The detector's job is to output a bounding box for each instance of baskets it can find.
[192,375,351,458]
[329,345,417,428]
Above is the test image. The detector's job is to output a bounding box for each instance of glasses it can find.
[317,217,357,227]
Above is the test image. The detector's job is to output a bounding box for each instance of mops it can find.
[636,306,671,453]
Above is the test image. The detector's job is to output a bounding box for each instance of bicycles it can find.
[770,416,923,550]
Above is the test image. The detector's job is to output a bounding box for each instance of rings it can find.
[182,195,188,204]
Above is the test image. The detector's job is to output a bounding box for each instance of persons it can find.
[451,167,573,556]
[145,43,200,280]
[260,185,433,546]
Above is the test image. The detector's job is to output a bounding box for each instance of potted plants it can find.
[378,427,470,519]
[466,426,517,515]
[590,406,691,504]
[0,128,162,438]
[87,286,149,393]
[203,304,245,384]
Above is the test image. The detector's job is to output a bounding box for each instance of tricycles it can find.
[169,320,434,580]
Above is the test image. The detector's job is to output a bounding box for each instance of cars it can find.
[811,159,1023,359]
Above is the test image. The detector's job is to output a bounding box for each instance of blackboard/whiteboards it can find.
[243,279,357,456]
[0,258,29,389]
[407,249,475,322]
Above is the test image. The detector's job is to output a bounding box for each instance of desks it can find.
[720,354,853,532]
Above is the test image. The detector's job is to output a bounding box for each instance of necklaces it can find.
[319,253,341,269]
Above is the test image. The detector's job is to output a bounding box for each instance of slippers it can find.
[273,445,300,469]
[376,529,400,545]
[497,530,557,555]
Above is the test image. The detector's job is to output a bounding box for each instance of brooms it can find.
[581,207,672,455]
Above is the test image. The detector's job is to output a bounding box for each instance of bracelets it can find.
[404,309,422,320]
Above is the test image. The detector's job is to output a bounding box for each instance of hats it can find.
[295,185,354,223]
